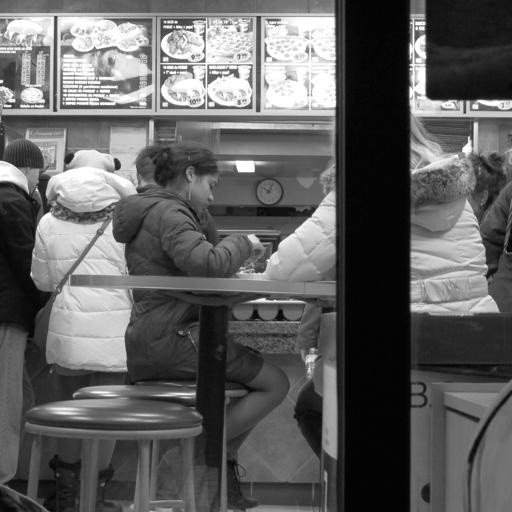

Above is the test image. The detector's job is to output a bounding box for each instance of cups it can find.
[192,65,205,83]
[238,65,251,80]
[193,20,205,39]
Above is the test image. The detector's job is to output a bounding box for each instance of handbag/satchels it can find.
[24,297,55,368]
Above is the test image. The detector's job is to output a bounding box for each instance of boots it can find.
[227,460,259,508]
[43,454,123,512]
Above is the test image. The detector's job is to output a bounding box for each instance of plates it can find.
[70,19,141,52]
[161,84,205,106]
[208,79,252,106]
[415,34,426,60]
[161,31,204,60]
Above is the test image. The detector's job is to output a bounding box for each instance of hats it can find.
[2,138,45,169]
[64,148,122,174]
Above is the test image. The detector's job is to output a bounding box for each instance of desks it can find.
[66,268,341,509]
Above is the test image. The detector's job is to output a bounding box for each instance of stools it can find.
[21,374,247,510]
